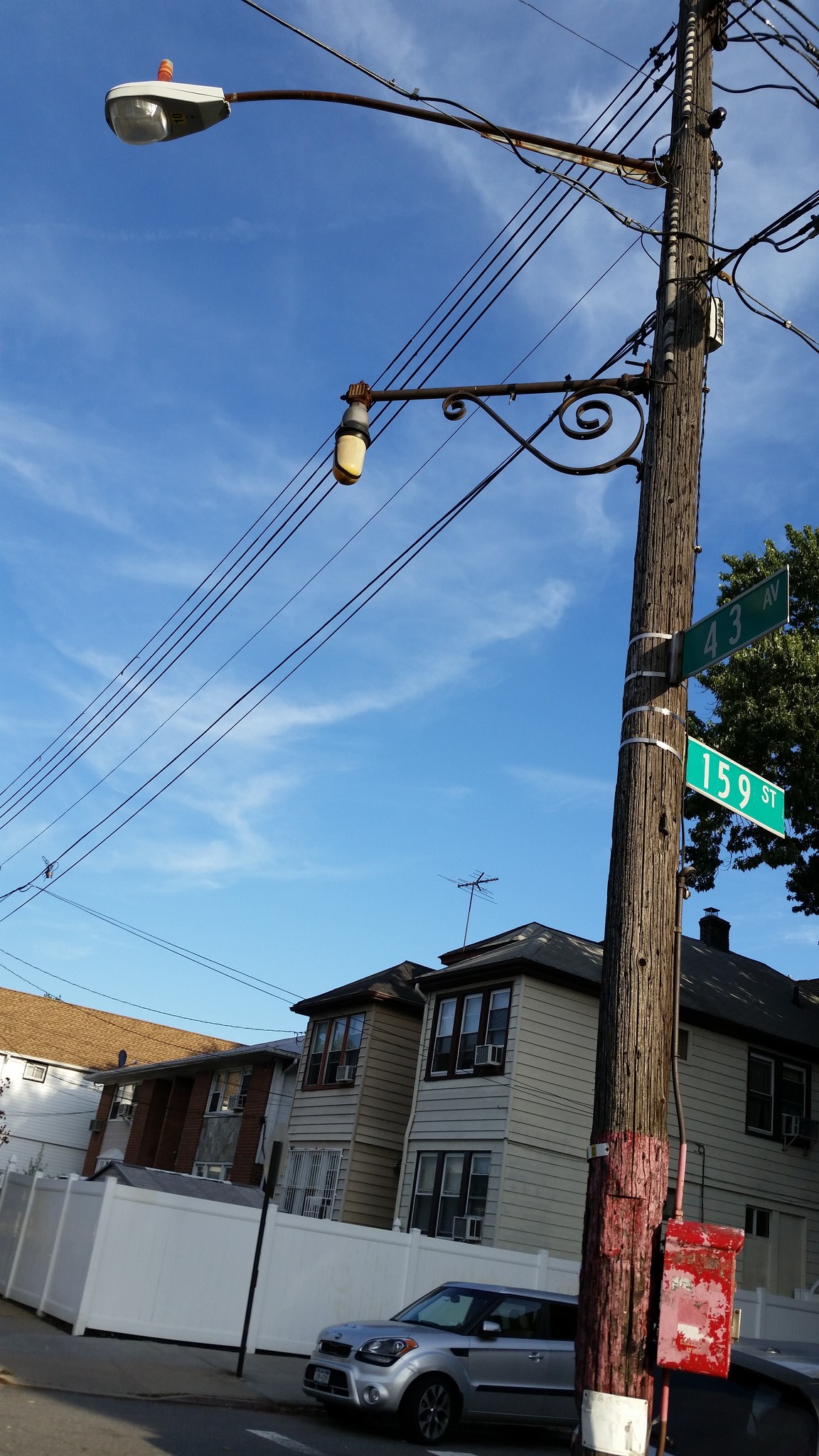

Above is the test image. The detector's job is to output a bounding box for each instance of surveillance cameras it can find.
[332,401,371,487]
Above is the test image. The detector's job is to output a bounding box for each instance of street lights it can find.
[99,59,722,1456]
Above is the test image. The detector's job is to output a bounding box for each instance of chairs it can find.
[503,1309,526,1338]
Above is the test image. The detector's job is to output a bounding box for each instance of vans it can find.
[302,1281,581,1450]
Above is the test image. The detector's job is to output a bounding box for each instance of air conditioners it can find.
[227,1094,244,1110]
[336,1065,356,1083]
[89,1119,103,1131]
[452,1216,484,1240]
[782,1115,819,1143]
[474,1044,504,1066]
[118,1104,136,1117]
